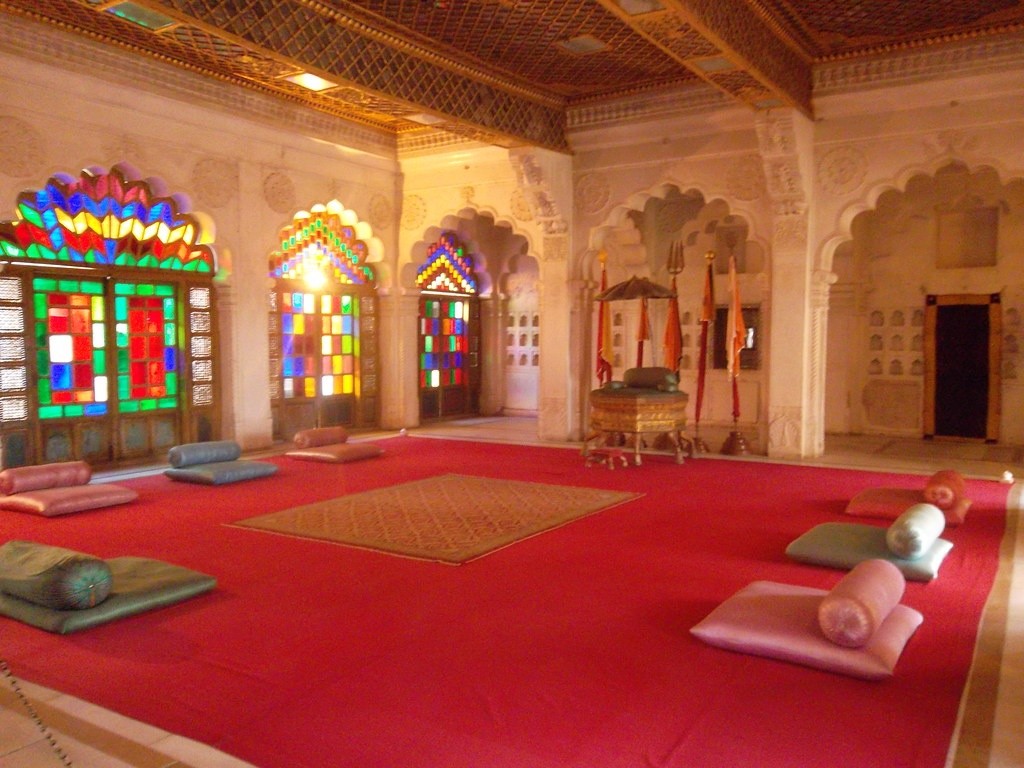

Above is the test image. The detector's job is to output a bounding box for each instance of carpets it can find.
[223,472,647,567]
[0,436,1016,768]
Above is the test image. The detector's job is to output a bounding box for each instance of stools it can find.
[583,447,629,470]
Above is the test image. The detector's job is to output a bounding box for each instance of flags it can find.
[635,294,653,343]
[725,254,752,378]
[698,259,717,324]
[596,270,616,388]
[662,271,685,373]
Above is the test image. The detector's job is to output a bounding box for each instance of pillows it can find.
[166,441,241,468]
[888,502,946,558]
[622,366,679,391]
[817,558,907,648]
[294,426,349,449]
[0,539,113,613]
[920,468,964,510]
[0,461,93,495]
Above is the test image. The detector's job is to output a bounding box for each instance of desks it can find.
[582,386,689,467]
